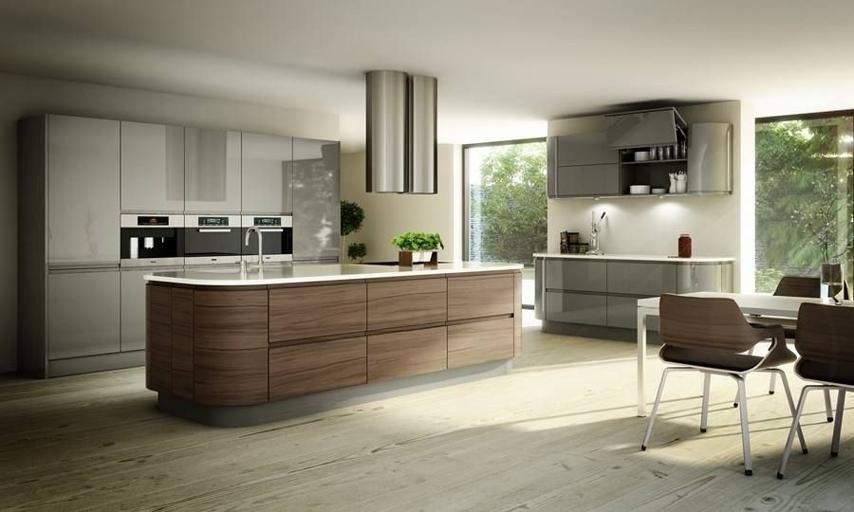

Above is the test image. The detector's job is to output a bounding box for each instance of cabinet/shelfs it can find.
[119,119,185,215]
[545,106,736,200]
[240,129,291,214]
[119,268,186,352]
[290,137,341,260]
[185,127,241,214]
[17,113,119,379]
[531,252,738,346]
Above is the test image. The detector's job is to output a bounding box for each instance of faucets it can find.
[244,226,265,265]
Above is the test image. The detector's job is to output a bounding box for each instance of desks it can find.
[635,292,854,484]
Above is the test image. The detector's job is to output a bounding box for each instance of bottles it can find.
[650,140,689,161]
[669,179,676,193]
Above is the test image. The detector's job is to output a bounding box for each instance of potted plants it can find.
[347,242,366,263]
[390,230,445,267]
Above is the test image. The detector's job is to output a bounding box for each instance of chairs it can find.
[734,275,823,408]
[776,301,854,480]
[639,293,809,475]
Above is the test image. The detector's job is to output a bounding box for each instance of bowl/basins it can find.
[634,151,650,161]
[629,184,651,195]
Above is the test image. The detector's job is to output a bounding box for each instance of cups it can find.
[398,251,412,267]
[677,180,687,194]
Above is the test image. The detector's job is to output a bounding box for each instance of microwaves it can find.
[120,212,184,268]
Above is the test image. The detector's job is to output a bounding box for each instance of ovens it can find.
[184,214,241,266]
[240,214,292,262]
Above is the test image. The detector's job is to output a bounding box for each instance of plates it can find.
[651,188,666,194]
